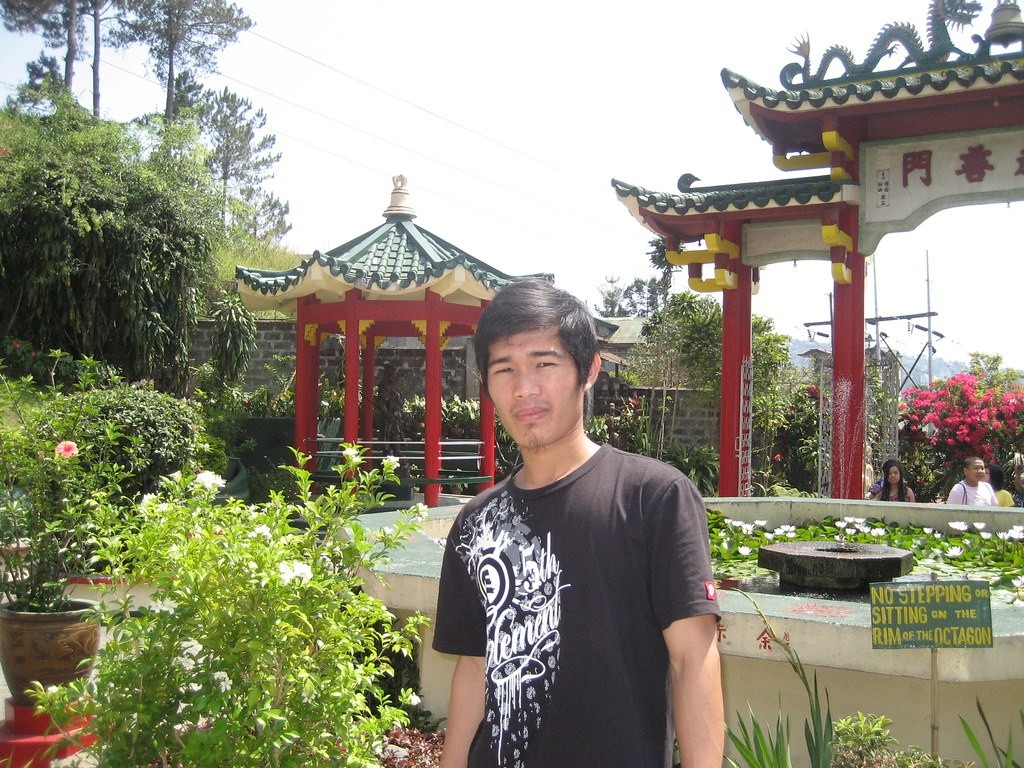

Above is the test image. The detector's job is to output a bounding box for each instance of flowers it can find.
[29,435,432,768]
[0,347,228,612]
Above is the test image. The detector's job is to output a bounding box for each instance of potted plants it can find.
[204,412,257,482]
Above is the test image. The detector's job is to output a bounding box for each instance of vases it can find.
[0,598,105,707]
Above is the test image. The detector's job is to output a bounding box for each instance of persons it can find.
[985,465,1015,507]
[432,277,724,768]
[869,460,915,502]
[947,456,999,506]
[378,364,405,458]
